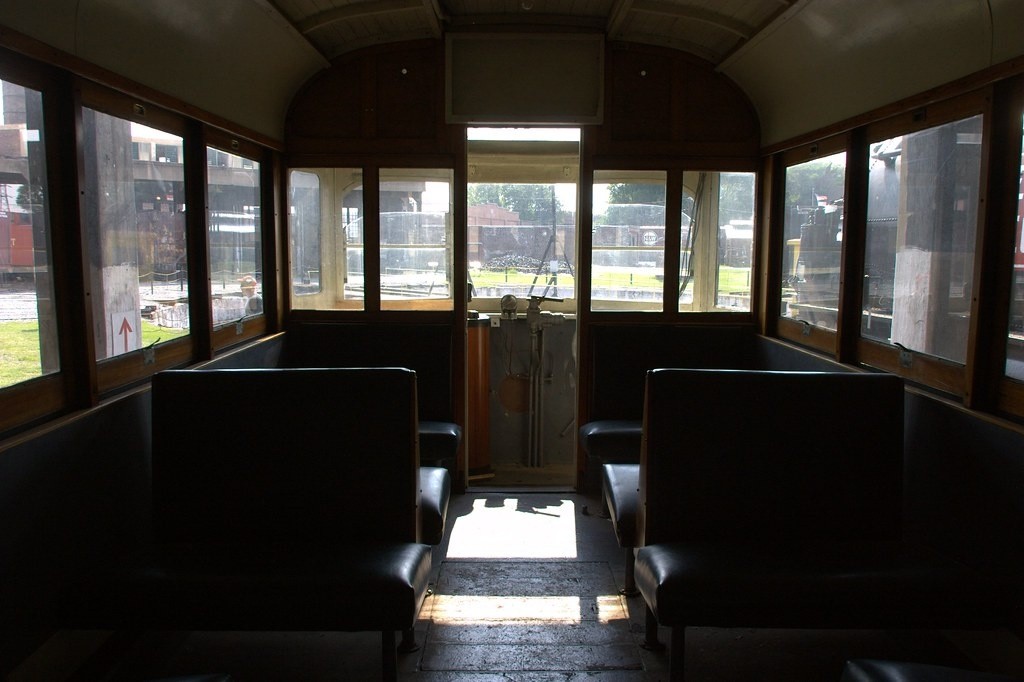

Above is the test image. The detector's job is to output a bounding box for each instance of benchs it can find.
[578,369,1023,681]
[46,367,464,681]
